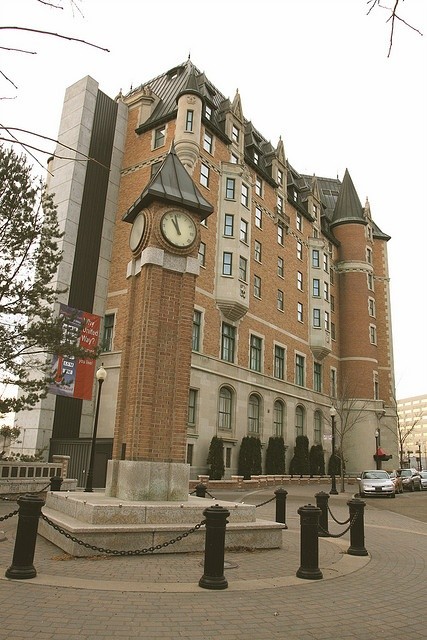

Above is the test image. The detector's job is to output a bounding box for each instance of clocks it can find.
[128,207,151,259]
[152,205,199,256]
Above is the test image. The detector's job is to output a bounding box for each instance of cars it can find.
[419,472,427,491]
[388,471,401,493]
[356,470,395,498]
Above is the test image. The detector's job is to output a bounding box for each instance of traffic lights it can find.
[373,454,391,461]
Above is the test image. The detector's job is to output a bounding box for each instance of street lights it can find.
[374,430,379,469]
[418,439,422,471]
[83,362,107,492]
[328,405,337,494]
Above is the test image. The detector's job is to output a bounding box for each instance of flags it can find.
[41,302,101,401]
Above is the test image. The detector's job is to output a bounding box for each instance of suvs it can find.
[396,469,422,492]
